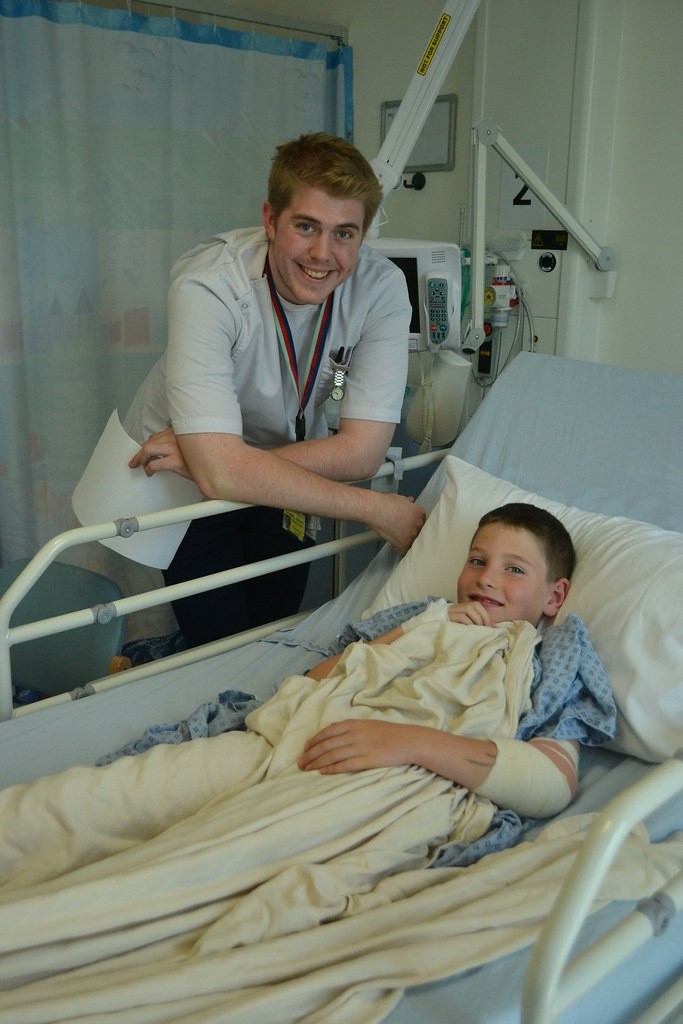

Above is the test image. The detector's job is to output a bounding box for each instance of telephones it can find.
[367,239,461,352]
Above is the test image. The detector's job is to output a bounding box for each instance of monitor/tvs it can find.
[365,237,461,352]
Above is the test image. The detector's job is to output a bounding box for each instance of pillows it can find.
[359,453,683,764]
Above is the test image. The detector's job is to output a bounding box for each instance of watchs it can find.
[328,368,347,405]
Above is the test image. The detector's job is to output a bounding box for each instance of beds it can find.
[1,350,682,1023]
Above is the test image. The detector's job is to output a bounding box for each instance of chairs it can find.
[1,556,125,696]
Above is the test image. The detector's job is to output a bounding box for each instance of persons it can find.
[118,131,428,666]
[297,503,585,820]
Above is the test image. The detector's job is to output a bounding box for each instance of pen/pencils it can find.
[335,346,343,365]
